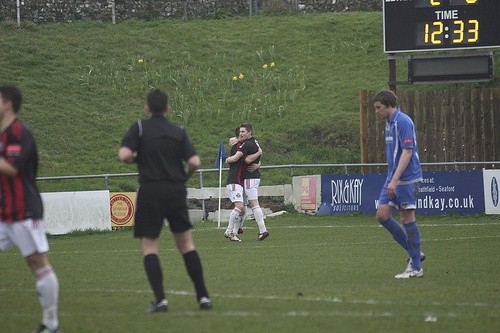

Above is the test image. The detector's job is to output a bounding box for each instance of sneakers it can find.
[394,268,424,278]
[231,236,241,242]
[238,228,243,233]
[405,251,425,271]
[199,296,212,309]
[224,230,230,238]
[256,232,269,240]
[145,298,169,312]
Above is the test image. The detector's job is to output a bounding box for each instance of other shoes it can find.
[36,322,61,333]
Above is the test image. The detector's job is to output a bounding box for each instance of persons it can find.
[0,85,59,333]
[373,90,426,277]
[224,124,270,242]
[118,89,213,309]
